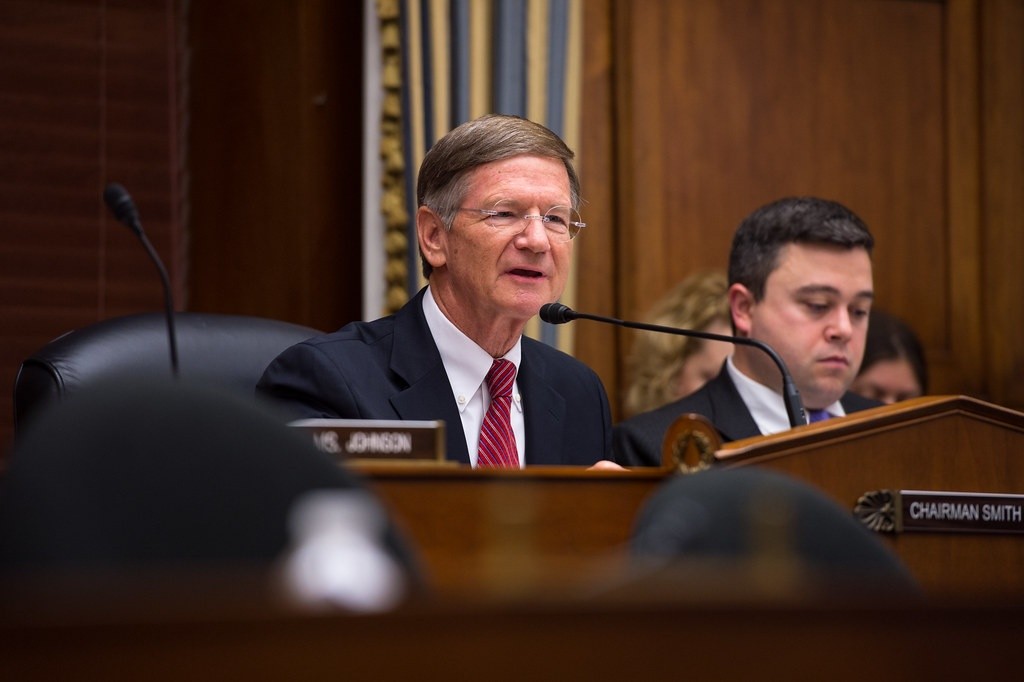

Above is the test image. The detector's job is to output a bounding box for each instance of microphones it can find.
[102,183,179,376]
[540,302,805,433]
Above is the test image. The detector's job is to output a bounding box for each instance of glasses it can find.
[454,197,586,244]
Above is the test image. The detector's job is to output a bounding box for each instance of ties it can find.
[809,407,830,424]
[474,360,520,469]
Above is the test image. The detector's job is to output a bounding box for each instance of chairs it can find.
[629,471,923,598]
[12,308,340,406]
[1,396,427,594]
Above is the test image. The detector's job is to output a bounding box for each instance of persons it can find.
[849,307,930,404]
[605,196,889,469]
[252,112,631,469]
